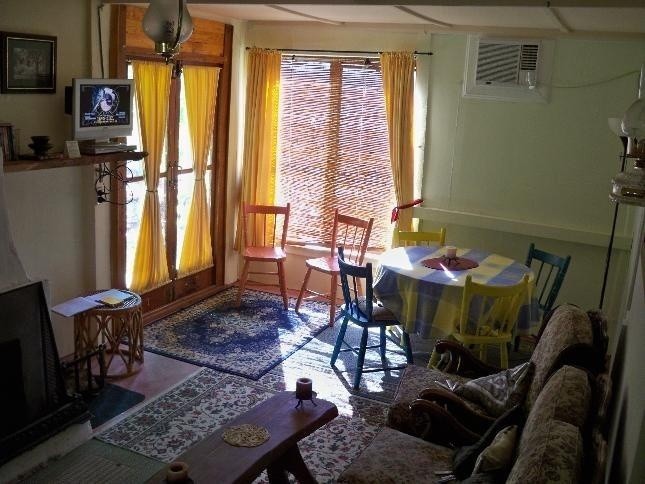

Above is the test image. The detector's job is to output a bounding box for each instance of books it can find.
[50,289,134,319]
[0,122,15,163]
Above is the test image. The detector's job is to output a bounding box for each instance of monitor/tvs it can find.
[66,78,136,152]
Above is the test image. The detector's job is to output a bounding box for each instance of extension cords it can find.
[97,181,106,205]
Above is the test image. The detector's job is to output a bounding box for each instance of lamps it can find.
[610,94,645,207]
[599,116,625,322]
[140,0,195,61]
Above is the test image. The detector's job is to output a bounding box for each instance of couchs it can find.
[389,300,613,420]
[342,365,603,481]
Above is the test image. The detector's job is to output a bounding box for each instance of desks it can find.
[76,285,147,380]
[136,389,341,484]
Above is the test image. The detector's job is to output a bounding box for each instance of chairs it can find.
[329,246,416,390]
[452,274,529,369]
[393,226,448,250]
[293,209,374,325]
[235,204,294,309]
[515,241,573,349]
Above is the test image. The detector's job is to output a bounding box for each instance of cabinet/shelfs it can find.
[142,266,219,317]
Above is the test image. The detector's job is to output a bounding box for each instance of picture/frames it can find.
[2,31,58,93]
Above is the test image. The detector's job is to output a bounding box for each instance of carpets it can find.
[121,283,346,380]
[96,369,380,483]
[264,311,415,403]
[19,439,165,483]
[68,340,199,432]
